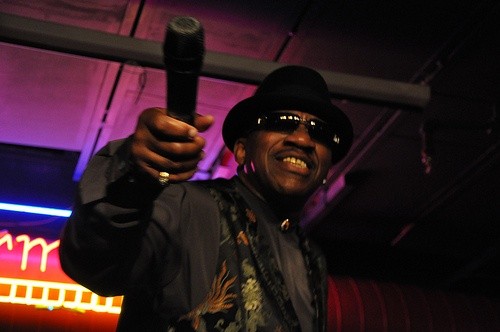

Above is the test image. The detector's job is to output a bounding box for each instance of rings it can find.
[158,170,170,185]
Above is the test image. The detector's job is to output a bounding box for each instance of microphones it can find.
[164,17,204,164]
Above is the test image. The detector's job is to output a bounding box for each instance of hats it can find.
[222,65,353,164]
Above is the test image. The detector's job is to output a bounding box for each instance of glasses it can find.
[242,112,333,149]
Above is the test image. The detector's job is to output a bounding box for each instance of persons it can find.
[57,64,353,332]
